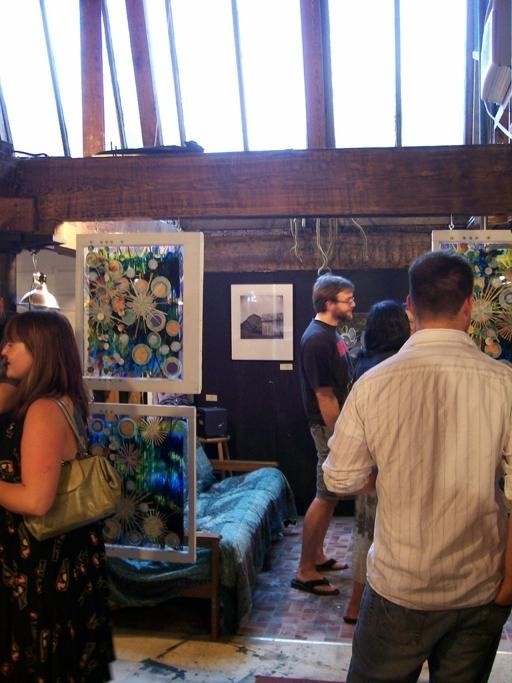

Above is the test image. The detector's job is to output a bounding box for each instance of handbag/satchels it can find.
[19,397,125,541]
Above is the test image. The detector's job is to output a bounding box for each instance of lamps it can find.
[230,282,294,360]
[17,269,60,311]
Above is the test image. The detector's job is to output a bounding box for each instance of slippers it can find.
[316,558,350,571]
[290,575,340,596]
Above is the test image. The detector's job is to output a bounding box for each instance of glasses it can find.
[337,297,356,304]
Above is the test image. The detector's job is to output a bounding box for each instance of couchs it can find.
[96,434,301,639]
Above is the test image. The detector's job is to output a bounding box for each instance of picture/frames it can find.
[432,228,512,369]
[89,403,195,564]
[74,232,204,391]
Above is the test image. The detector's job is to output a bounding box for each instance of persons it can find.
[343,300,410,624]
[0,311,116,683]
[290,273,357,596]
[0,297,20,415]
[320,250,512,683]
